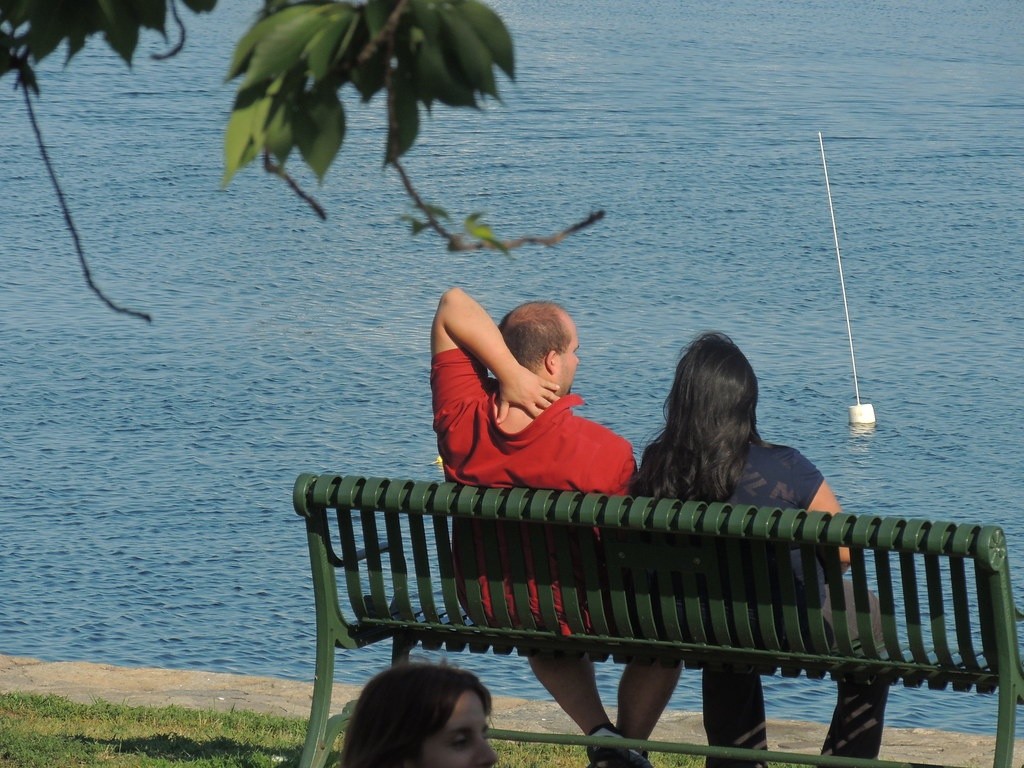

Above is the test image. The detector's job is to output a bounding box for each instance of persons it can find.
[344,663,497,768]
[626,333,890,768]
[429,288,652,768]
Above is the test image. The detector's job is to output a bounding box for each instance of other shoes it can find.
[585,722,654,768]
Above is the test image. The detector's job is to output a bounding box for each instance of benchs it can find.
[291,471,1024,768]
